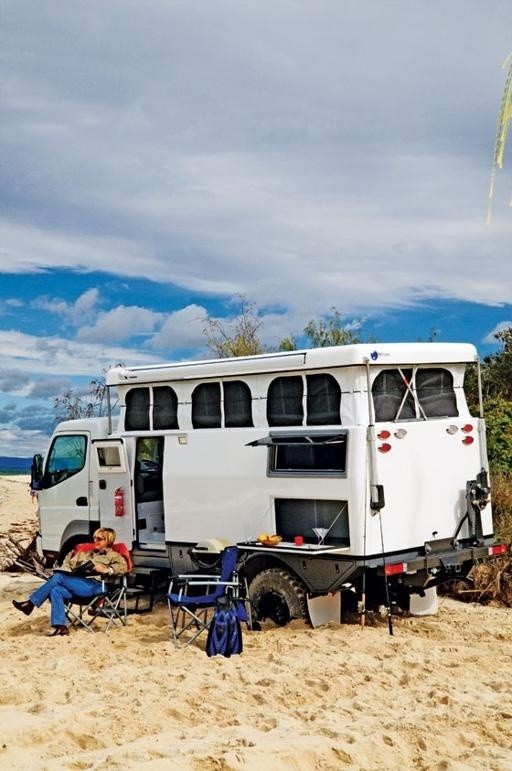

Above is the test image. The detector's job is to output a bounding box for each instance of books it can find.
[52,559,103,579]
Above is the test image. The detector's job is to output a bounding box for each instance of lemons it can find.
[258,534,267,541]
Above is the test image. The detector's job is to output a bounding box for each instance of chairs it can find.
[165,545,240,649]
[65,541,132,634]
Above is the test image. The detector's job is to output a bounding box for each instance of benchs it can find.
[127,566,161,612]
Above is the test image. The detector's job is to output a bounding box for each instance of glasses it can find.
[93,536,106,541]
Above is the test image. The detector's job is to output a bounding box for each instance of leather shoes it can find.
[13,599,34,615]
[47,626,68,636]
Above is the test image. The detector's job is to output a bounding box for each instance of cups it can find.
[294,536,303,545]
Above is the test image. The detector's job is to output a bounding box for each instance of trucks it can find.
[30,341,506,625]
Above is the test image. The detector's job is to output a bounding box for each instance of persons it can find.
[12,525,129,637]
